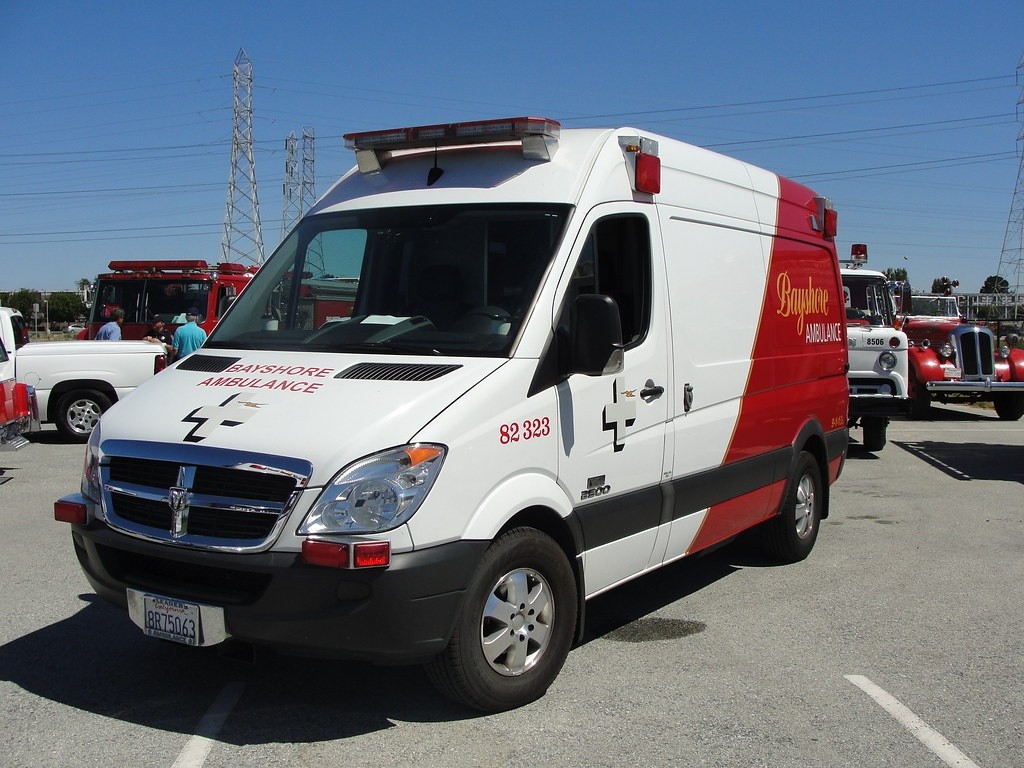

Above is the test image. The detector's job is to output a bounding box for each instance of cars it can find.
[896,294,1023,419]
[61,323,86,334]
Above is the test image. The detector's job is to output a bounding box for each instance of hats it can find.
[152,317,162,325]
[187,307,199,316]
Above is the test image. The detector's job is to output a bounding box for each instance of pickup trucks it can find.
[0,304,172,445]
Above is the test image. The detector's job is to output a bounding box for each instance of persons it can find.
[94,309,125,340]
[143,307,207,366]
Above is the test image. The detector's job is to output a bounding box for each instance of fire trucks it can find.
[832,242,912,455]
[75,258,362,370]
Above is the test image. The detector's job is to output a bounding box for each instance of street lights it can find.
[42,300,50,335]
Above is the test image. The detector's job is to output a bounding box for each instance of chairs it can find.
[510,266,584,331]
[398,265,475,333]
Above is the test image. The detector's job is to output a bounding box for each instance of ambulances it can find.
[55,109,856,714]
